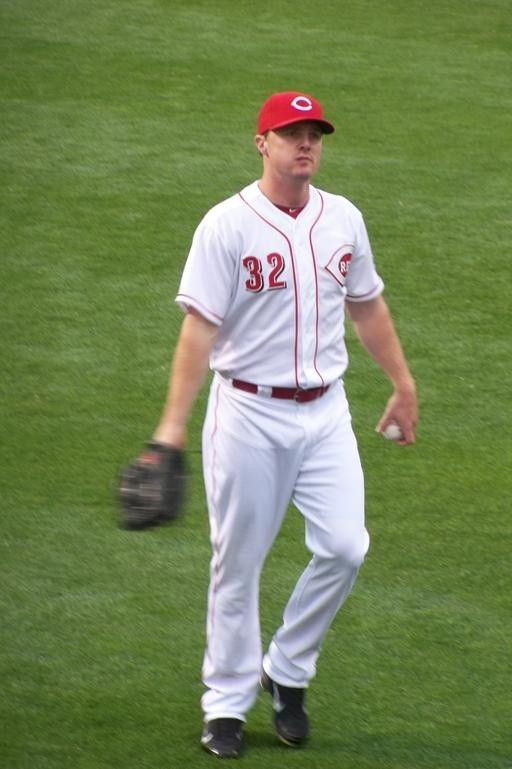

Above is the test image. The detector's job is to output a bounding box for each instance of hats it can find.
[257,90,336,136]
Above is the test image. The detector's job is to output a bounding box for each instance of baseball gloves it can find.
[114,443,186,532]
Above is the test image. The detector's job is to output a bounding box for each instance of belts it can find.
[232,379,330,403]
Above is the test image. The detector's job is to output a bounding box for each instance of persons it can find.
[116,90,420,759]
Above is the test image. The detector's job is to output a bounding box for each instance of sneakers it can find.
[201,717,243,760]
[259,670,310,748]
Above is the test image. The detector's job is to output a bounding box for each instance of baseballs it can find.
[380,416,405,443]
